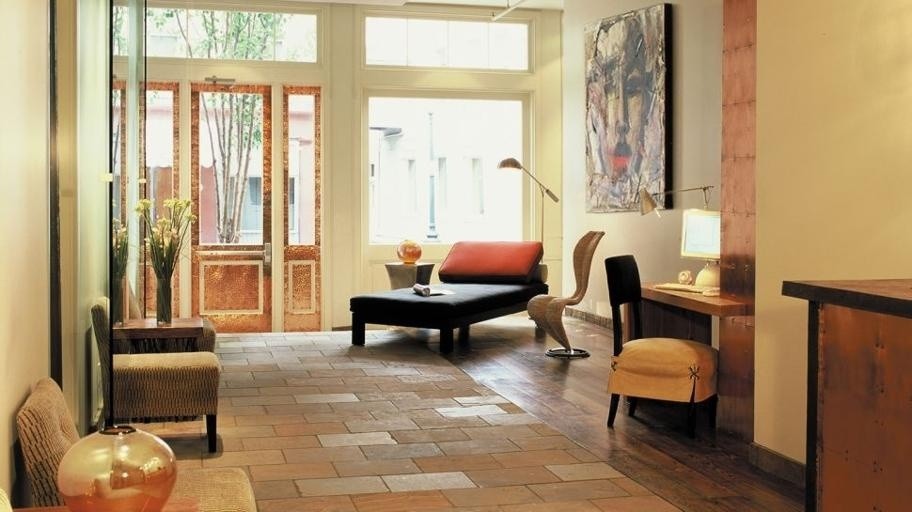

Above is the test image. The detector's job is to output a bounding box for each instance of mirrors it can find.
[111,1,131,322]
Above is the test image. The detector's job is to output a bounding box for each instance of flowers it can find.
[137,197,198,278]
[110,220,129,276]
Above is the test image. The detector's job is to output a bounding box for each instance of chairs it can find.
[16,378,257,512]
[127,283,216,352]
[92,295,220,452]
[527,230,605,358]
[604,255,719,431]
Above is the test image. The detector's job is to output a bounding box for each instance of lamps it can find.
[498,158,559,264]
[639,186,721,289]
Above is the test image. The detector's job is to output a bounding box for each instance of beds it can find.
[350,240,549,355]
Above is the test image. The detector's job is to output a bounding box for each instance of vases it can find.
[112,275,127,320]
[157,277,173,325]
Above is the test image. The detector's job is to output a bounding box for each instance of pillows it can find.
[438,241,544,282]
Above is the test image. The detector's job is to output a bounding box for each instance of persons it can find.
[587,11,653,213]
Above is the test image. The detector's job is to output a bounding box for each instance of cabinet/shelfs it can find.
[781,280,912,512]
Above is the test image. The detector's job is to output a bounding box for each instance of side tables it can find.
[113,319,208,352]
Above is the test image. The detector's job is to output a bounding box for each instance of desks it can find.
[622,282,744,404]
